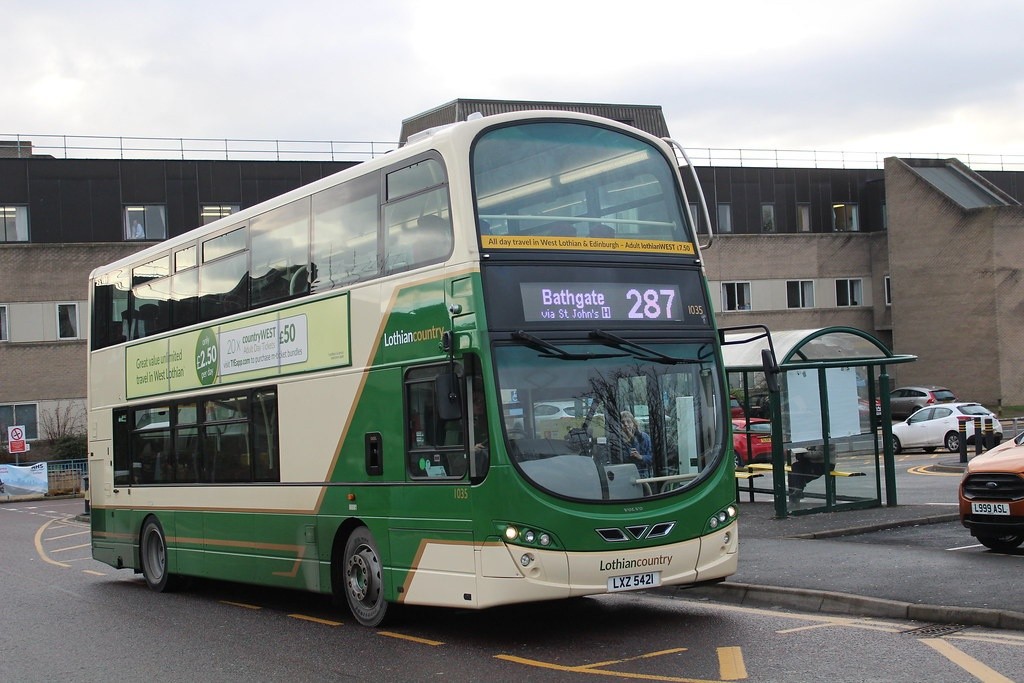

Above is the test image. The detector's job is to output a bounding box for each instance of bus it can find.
[85,112,782,628]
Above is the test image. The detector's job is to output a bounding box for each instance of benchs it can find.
[735,471,763,479]
[748,464,867,477]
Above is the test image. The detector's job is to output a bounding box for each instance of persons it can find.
[788,439,836,509]
[472,390,489,454]
[132,219,145,239]
[620,412,653,479]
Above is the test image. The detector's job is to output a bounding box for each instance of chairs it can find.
[413,213,616,261]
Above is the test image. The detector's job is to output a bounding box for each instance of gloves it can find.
[796,453,803,460]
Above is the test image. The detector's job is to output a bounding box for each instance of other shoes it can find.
[789,485,804,491]
[787,501,800,511]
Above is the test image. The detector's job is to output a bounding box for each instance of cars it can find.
[625,405,671,436]
[729,396,745,418]
[746,384,882,424]
[958,431,1024,551]
[890,386,962,419]
[513,401,606,439]
[891,401,1003,452]
[733,419,772,470]
